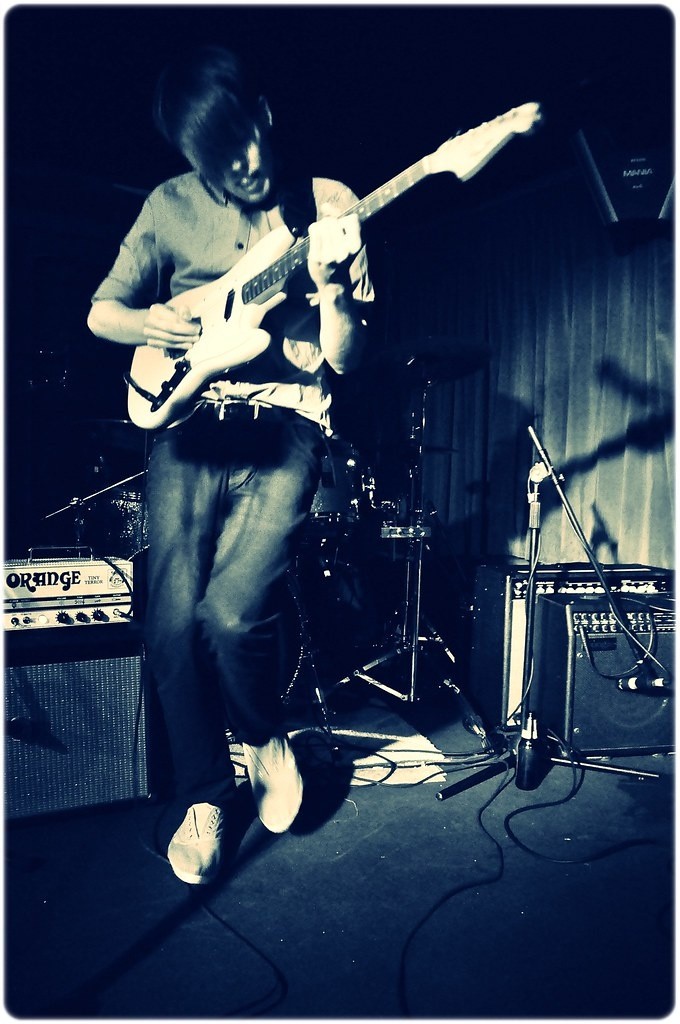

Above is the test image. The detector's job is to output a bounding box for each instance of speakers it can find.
[502,564,674,732]
[4,621,151,821]
[528,591,674,759]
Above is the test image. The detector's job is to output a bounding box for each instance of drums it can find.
[80,482,154,559]
[127,542,315,744]
[300,449,362,539]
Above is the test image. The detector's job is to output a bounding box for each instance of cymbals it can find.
[68,418,155,448]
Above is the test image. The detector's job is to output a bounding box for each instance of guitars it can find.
[123,99,543,434]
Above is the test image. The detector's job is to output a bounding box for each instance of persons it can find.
[87,43,376,885]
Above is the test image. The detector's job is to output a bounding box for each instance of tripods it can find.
[316,379,663,799]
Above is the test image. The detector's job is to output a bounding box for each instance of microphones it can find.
[616,678,671,692]
